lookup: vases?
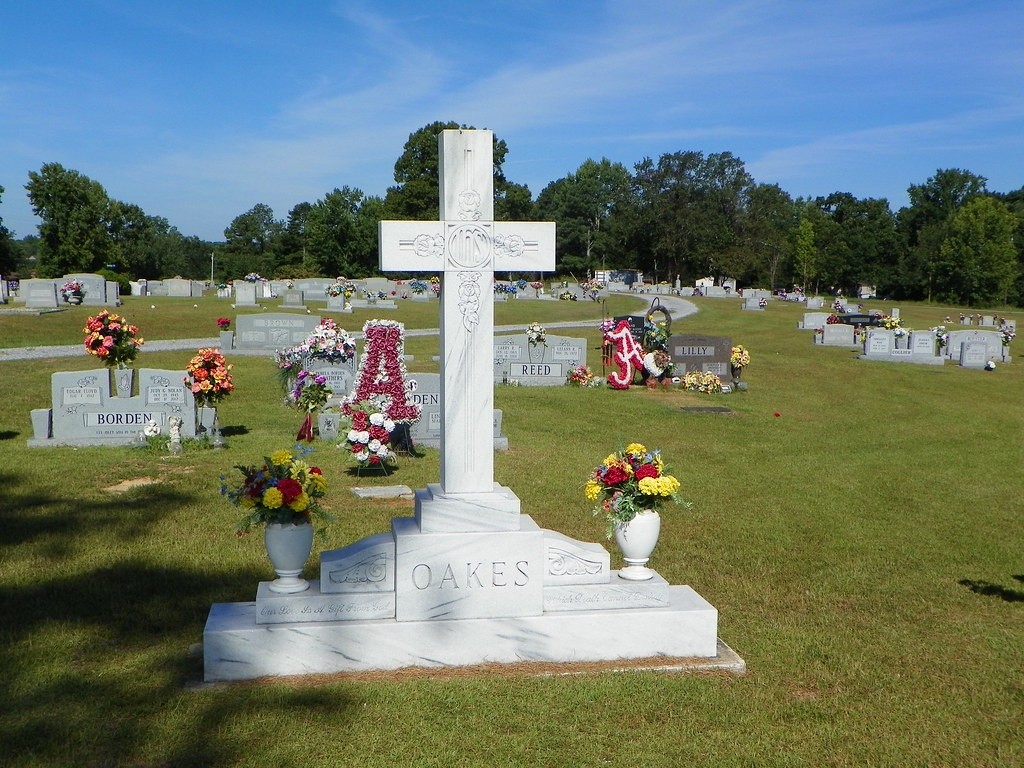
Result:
[731,365,741,378]
[197,407,217,436]
[68,296,80,306]
[939,346,947,356]
[30,408,53,439]
[615,507,660,580]
[220,330,234,350]
[896,337,909,349]
[318,413,340,440]
[114,369,134,397]
[854,335,862,343]
[286,379,297,391]
[1002,346,1010,357]
[264,522,315,594]
[814,334,823,344]
[530,342,545,364]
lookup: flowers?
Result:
[324,276,357,311]
[219,284,226,288]
[431,276,440,295]
[82,310,146,370]
[409,278,428,293]
[401,292,408,299]
[391,290,396,296]
[580,278,605,304]
[244,273,268,283]
[286,281,294,289]
[944,316,954,323]
[529,281,544,289]
[218,444,337,543]
[661,281,668,285]
[759,297,767,306]
[565,361,593,388]
[779,288,787,297]
[987,361,997,370]
[681,370,722,395]
[826,314,843,324]
[271,317,356,391]
[959,313,982,320]
[794,286,805,295]
[814,328,824,334]
[929,326,949,347]
[339,319,423,466]
[494,277,527,295]
[59,279,84,296]
[217,318,231,329]
[598,315,680,391]
[873,313,913,338]
[996,317,1017,346]
[579,438,695,542]
[362,289,387,300]
[833,300,845,312]
[729,345,751,369]
[560,291,578,301]
[854,322,871,342]
[182,347,234,407]
[523,321,548,347]
[281,371,332,413]
